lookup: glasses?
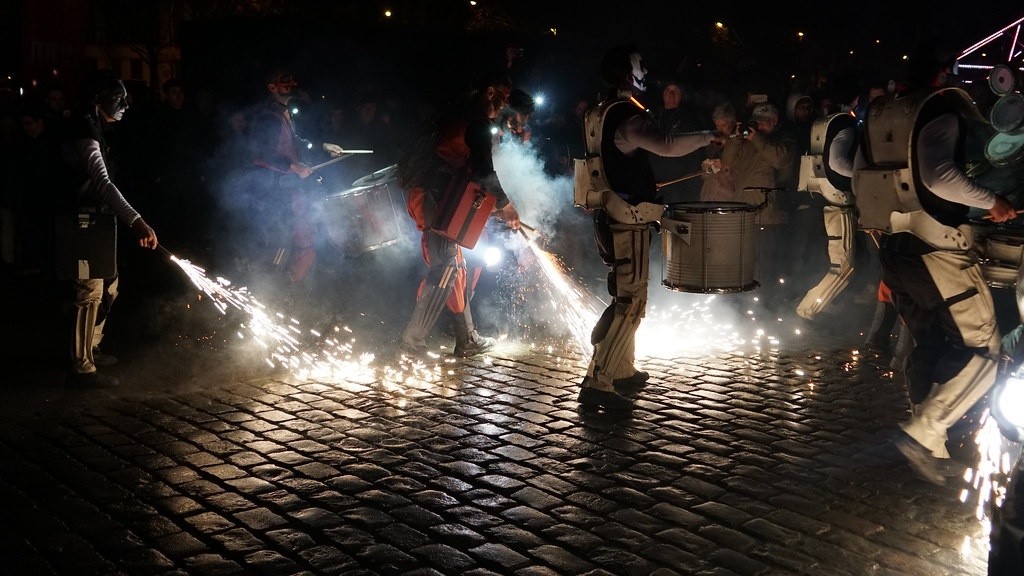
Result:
[796,105,811,111]
[22,120,37,128]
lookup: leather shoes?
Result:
[580,387,633,414]
[889,429,947,487]
[913,453,971,482]
[612,369,649,387]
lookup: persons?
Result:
[852,38,1003,489]
[983,262,1024,576]
[575,43,730,413]
[858,228,916,374]
[398,74,522,359]
[0,0,1024,311]
[64,76,160,391]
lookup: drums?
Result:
[315,165,418,257]
[965,215,1023,288]
[663,201,763,295]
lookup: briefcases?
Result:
[430,174,497,250]
[10,207,119,285]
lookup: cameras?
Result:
[736,121,759,140]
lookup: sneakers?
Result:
[395,343,447,367]
[455,332,498,357]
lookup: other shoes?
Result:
[69,373,120,390]
[90,354,119,368]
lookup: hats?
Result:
[256,70,297,89]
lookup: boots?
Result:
[866,301,896,359]
[891,325,914,371]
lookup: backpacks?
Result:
[396,119,446,230]
